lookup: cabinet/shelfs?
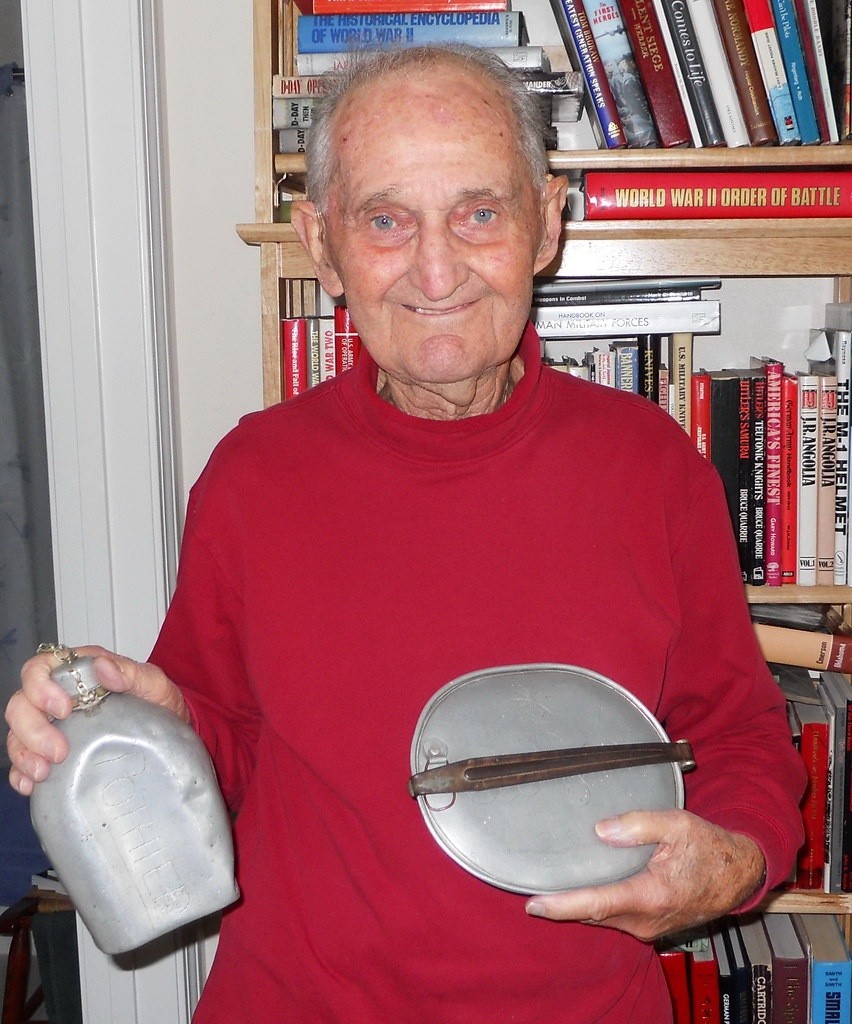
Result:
[236,0,852,1024]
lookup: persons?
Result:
[4,39,806,1024]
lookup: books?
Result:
[265,0,852,1024]
[26,868,67,894]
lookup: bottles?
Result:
[31,657,242,955]
